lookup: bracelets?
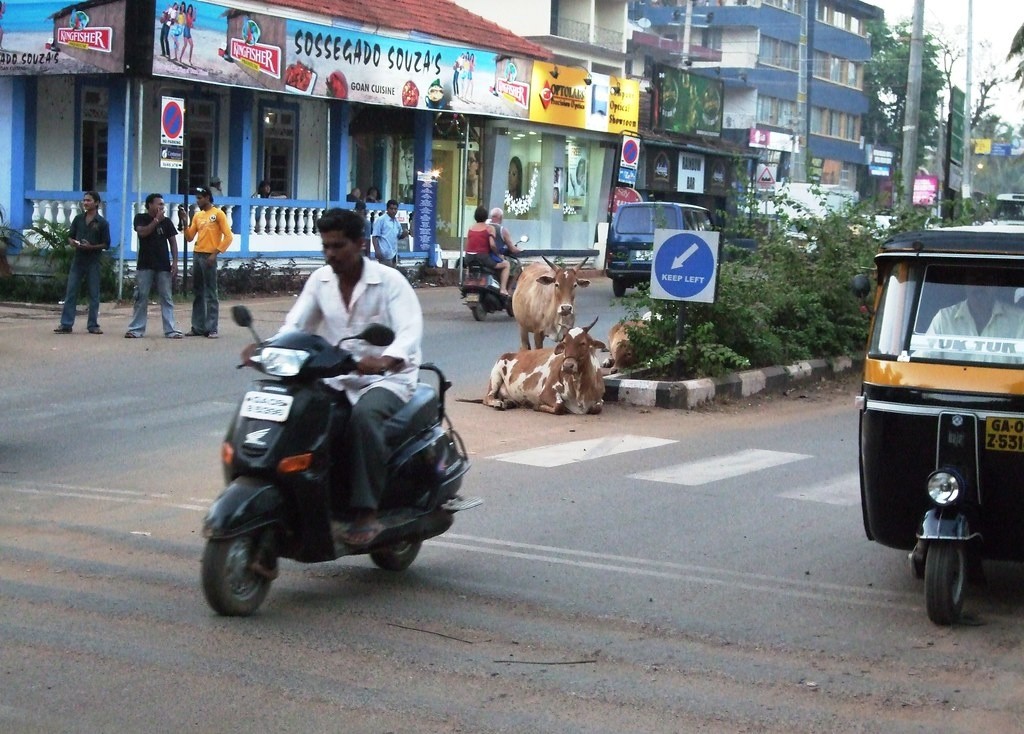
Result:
[154,218,159,224]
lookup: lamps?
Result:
[684,59,692,67]
[740,74,746,80]
[611,76,620,93]
[714,66,720,73]
[568,63,593,85]
[550,65,559,79]
[629,75,656,93]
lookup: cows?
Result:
[509,253,592,350]
[454,315,609,416]
[600,318,665,375]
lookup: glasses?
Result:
[196,188,207,193]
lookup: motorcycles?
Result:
[852,227,1024,625]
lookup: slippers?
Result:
[343,524,384,545]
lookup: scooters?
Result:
[201,305,485,614]
[458,234,531,322]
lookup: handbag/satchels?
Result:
[490,252,503,263]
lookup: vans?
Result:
[603,201,718,297]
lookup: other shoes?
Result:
[174,333,183,339]
[54,325,72,334]
[124,333,136,338]
[185,330,195,336]
[208,331,218,339]
[91,328,103,334]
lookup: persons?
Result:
[923,266,1024,339]
[508,156,522,200]
[554,188,559,205]
[179,187,234,338]
[125,194,182,339]
[355,202,371,258]
[347,189,361,202]
[54,190,111,334]
[372,199,409,267]
[488,208,523,263]
[179,5,196,66]
[453,52,475,102]
[366,188,380,203]
[467,206,511,297]
[160,2,178,58]
[208,176,223,197]
[172,2,185,61]
[240,208,423,547]
[253,181,286,199]
[466,151,480,198]
